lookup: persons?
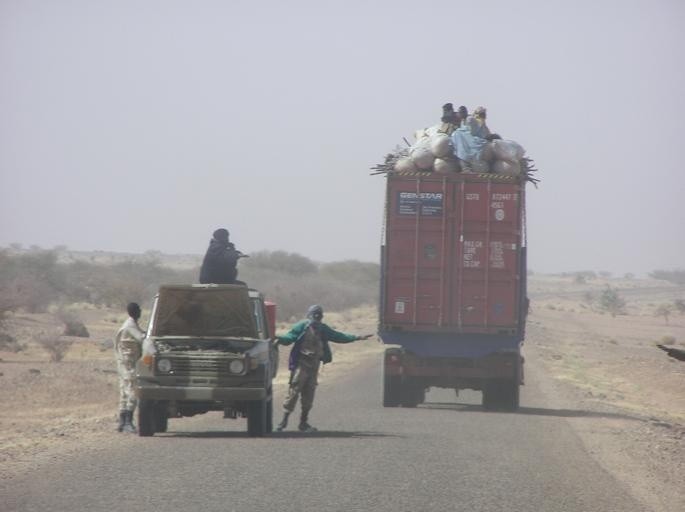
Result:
[272,304,375,433]
[467,106,490,136]
[198,228,250,286]
[441,104,461,125]
[457,106,469,119]
[112,300,145,434]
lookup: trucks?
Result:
[379,168,530,411]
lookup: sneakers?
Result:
[278,421,311,432]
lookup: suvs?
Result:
[134,280,280,437]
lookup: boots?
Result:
[117,410,136,433]
